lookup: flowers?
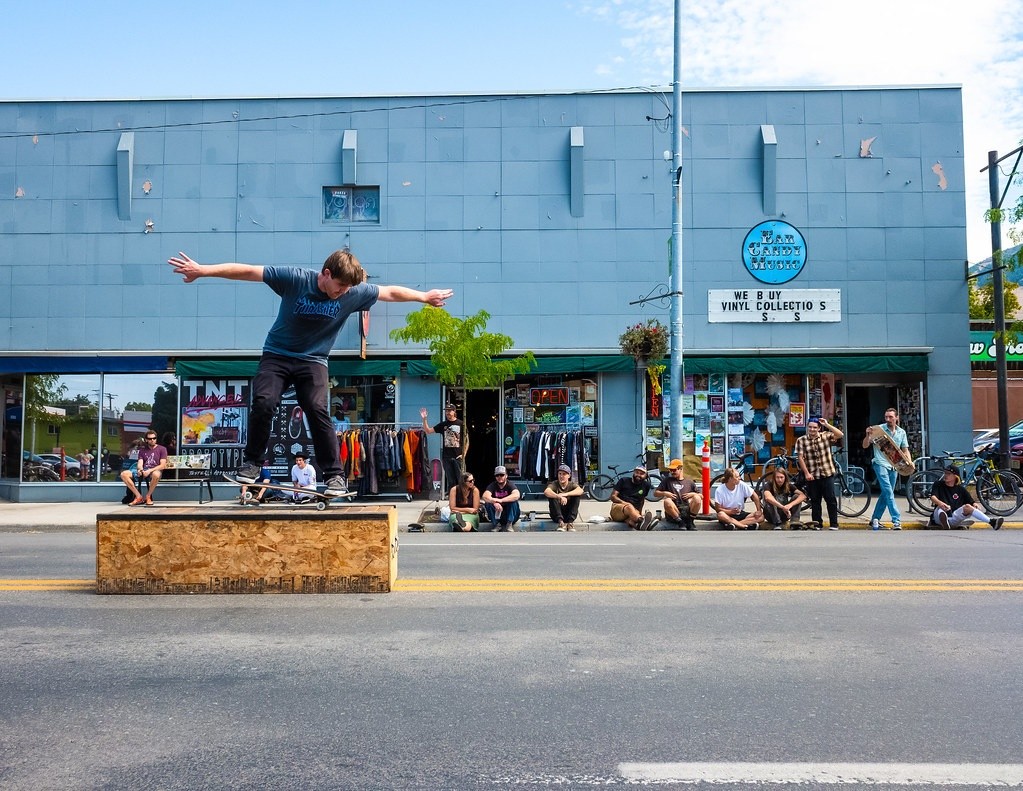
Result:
[619,320,666,360]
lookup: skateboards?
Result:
[915,518,975,530]
[801,521,820,531]
[407,523,426,532]
[868,425,915,476]
[790,523,803,530]
[519,510,550,522]
[222,472,358,512]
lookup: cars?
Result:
[22,449,139,482]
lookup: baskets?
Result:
[992,452,1010,470]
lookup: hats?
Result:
[667,459,683,469]
[557,465,572,474]
[634,465,646,474]
[293,452,309,460]
[494,466,508,474]
[808,418,818,422]
[442,404,457,410]
[946,466,961,475]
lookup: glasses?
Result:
[465,479,475,483]
[636,471,645,475]
[146,437,156,440]
[495,474,506,477]
[669,468,680,472]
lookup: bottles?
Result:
[645,509,649,514]
[736,503,741,515]
[140,470,143,481]
[873,519,879,531]
[435,500,439,515]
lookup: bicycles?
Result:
[708,441,872,517]
[904,417,1023,518]
[588,450,664,502]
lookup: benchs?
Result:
[132,454,215,503]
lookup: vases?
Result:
[636,338,654,355]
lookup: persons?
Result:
[167,250,453,495]
[863,408,911,530]
[483,466,521,532]
[449,472,480,532]
[101,443,110,476]
[74,449,94,480]
[762,467,806,530]
[793,418,845,530]
[239,468,273,503]
[88,444,97,478]
[162,432,177,456]
[931,465,1005,530]
[654,458,703,531]
[120,430,167,505]
[544,465,583,531]
[420,404,469,490]
[715,468,764,530]
[292,452,316,504]
[610,465,659,531]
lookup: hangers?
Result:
[331,423,424,436]
[527,420,583,432]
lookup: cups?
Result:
[656,510,661,521]
[521,520,531,532]
[539,521,545,531]
[530,511,535,522]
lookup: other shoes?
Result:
[635,511,902,530]
[456,512,467,527]
[938,512,951,530]
[557,524,566,531]
[507,523,514,532]
[129,497,154,505]
[452,522,462,532]
[491,523,502,532]
[989,517,1004,530]
[567,523,574,531]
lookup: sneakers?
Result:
[324,475,349,496]
[235,461,261,484]
